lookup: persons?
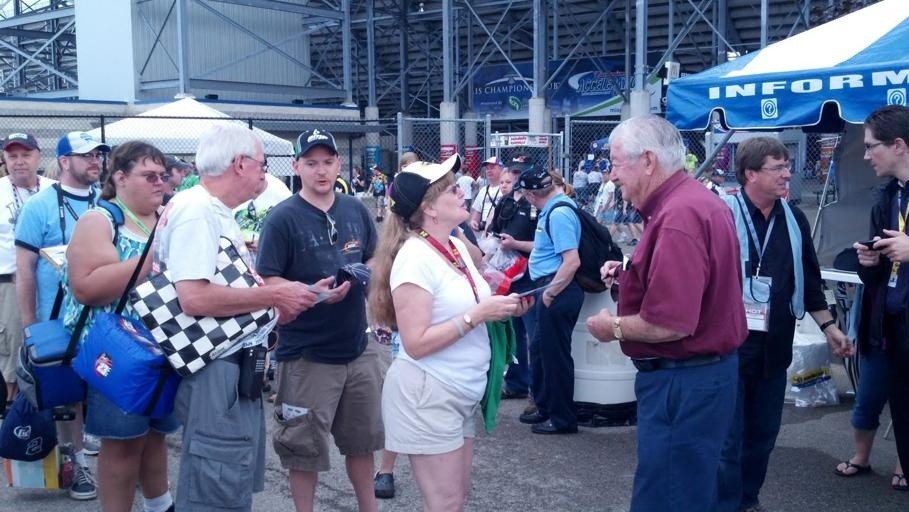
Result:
[1,124,586,512]
[717,136,855,511]
[831,105,909,492]
[569,145,731,246]
[585,114,749,511]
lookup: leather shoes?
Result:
[501,386,578,435]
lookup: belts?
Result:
[631,354,720,370]
[535,274,555,287]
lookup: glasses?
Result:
[64,152,101,161]
[233,155,268,172]
[863,143,882,152]
[144,176,169,183]
[756,164,790,173]
[445,184,460,194]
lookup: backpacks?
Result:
[546,201,624,292]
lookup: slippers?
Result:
[891,474,908,489]
[833,460,871,476]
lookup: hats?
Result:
[163,156,189,170]
[295,130,338,160]
[2,132,40,150]
[481,157,553,188]
[390,152,461,217]
[56,132,110,156]
[370,166,378,170]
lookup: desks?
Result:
[819,266,865,348]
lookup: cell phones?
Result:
[492,231,504,240]
[859,239,886,251]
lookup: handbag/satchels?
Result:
[0,312,180,462]
[237,346,265,402]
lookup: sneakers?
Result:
[374,470,395,497]
[68,433,100,500]
[618,232,638,246]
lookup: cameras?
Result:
[479,221,485,230]
